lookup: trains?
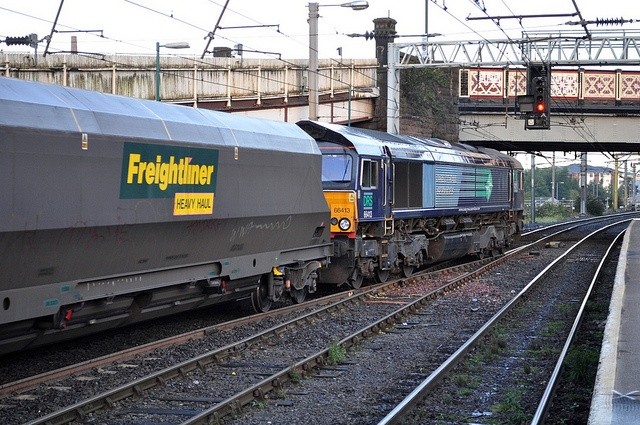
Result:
[0,70,527,359]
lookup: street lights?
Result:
[153,40,194,101]
[307,0,369,121]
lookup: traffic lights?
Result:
[523,62,550,130]
